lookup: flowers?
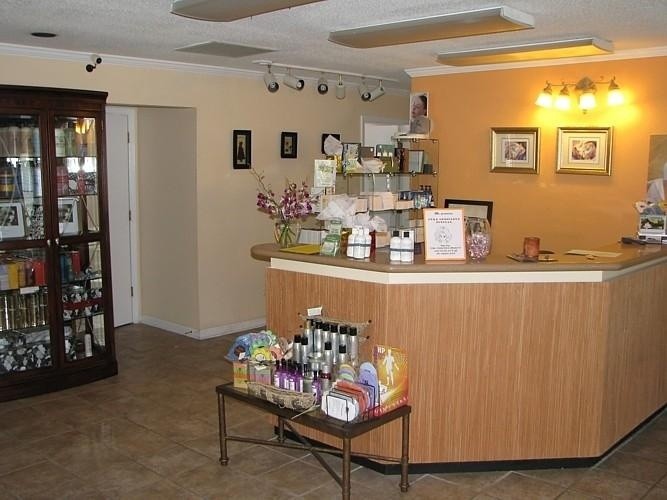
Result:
[251,167,321,250]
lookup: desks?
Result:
[215,380,411,500]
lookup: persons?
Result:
[2,208,15,226]
[64,206,72,222]
[473,222,482,235]
[410,94,427,120]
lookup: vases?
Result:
[274,219,302,249]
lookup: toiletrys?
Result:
[61,248,80,283]
[57,159,69,196]
[292,318,361,373]
[345,227,372,261]
[389,230,413,263]
[77,166,86,193]
[1,257,46,289]
[272,358,330,406]
[83,330,92,358]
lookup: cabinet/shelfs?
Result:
[0,83,118,405]
[335,138,438,231]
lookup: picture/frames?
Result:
[280,131,297,158]
[554,125,613,177]
[445,198,493,230]
[233,129,251,169]
[321,134,340,155]
[489,126,541,175]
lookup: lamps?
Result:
[327,6,536,49]
[436,39,614,67]
[536,75,624,109]
[261,62,386,103]
[170,0,324,22]
